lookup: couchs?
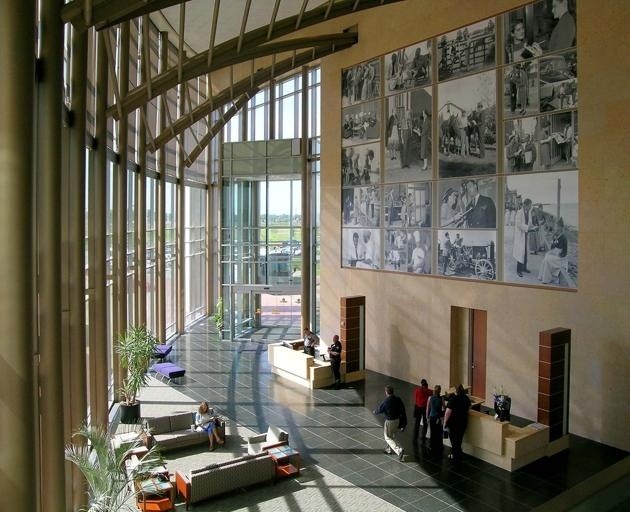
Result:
[141,408,225,452]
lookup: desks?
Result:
[263,441,301,482]
[135,475,173,512]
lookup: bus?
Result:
[258,246,293,286]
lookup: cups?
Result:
[191,424,195,432]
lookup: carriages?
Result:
[437,103,481,161]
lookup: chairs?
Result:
[247,424,289,456]
[125,454,170,492]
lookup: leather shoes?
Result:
[208,439,225,451]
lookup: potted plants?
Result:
[213,297,225,339]
[112,320,162,423]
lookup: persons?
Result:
[302,327,319,358]
[327,334,342,384]
[341,0,576,285]
[372,383,408,462]
[443,384,472,474]
[425,385,446,449]
[411,378,433,441]
[195,401,224,453]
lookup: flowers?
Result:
[491,385,506,410]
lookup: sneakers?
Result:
[398,448,406,462]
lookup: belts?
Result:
[387,417,400,420]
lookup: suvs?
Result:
[446,244,496,281]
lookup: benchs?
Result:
[152,362,186,385]
[149,345,172,362]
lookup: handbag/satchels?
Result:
[397,408,407,431]
[443,430,449,439]
